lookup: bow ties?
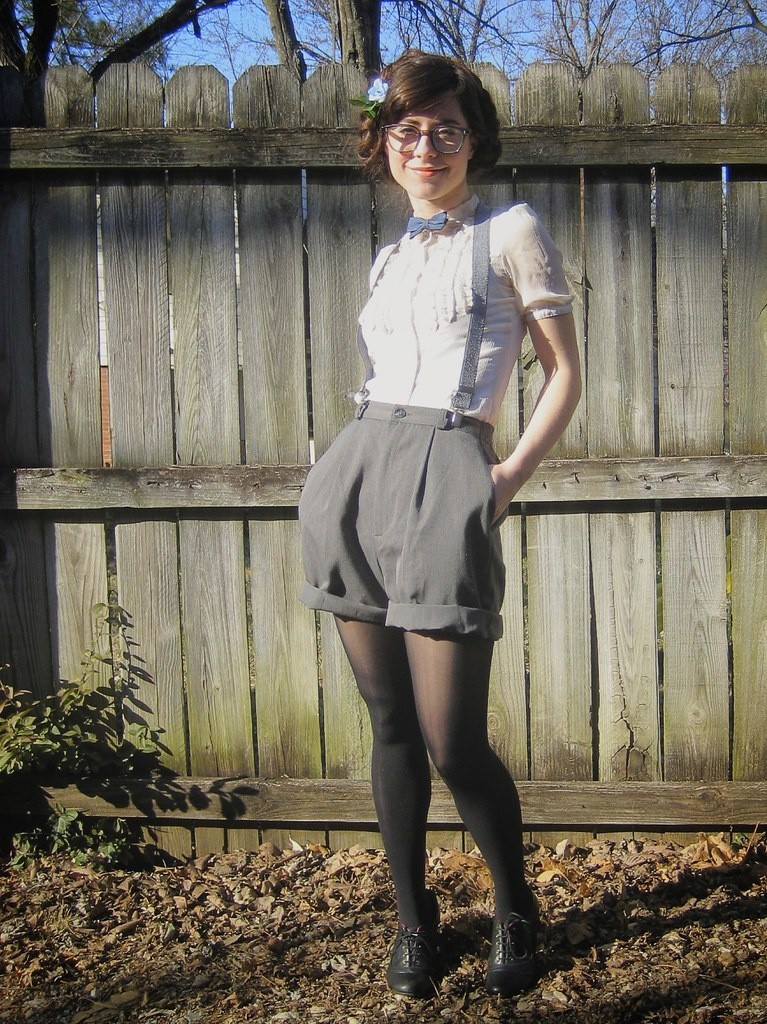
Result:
[407,211,448,240]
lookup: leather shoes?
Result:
[382,889,439,997]
[486,883,540,997]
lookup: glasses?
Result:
[382,124,472,154]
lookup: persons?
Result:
[296,52,581,998]
